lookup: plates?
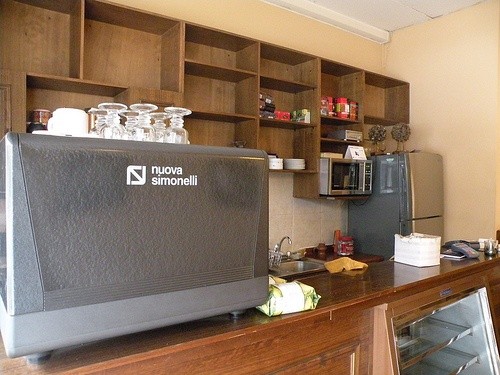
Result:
[284,159,306,170]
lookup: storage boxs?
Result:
[394,232,441,267]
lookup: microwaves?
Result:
[320,159,373,195]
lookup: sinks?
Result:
[280,257,328,276]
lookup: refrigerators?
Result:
[347,153,444,259]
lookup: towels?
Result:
[324,257,368,274]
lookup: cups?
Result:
[47,107,89,134]
[484,240,497,257]
[31,109,51,123]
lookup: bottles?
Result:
[27,119,44,133]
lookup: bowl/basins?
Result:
[268,158,284,170]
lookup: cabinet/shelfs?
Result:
[88,250,500,375]
[0,0,410,200]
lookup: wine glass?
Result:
[87,103,193,144]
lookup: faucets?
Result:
[278,235,293,254]
